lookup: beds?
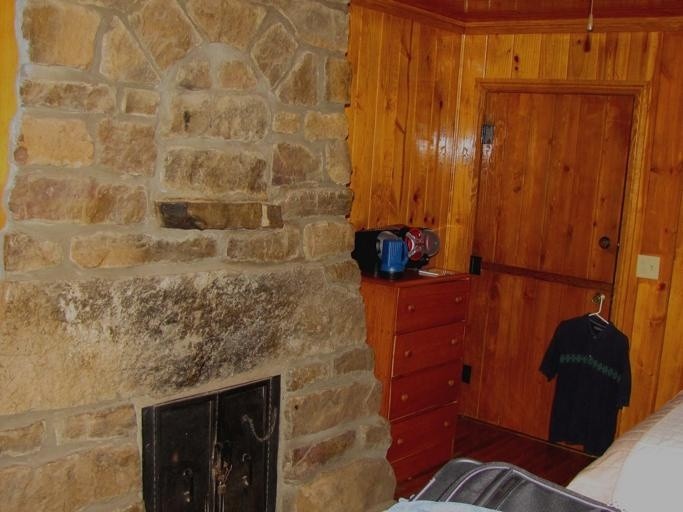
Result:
[567,388,683,512]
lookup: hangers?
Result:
[587,294,610,325]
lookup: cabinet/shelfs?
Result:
[358,278,469,500]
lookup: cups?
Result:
[382,239,407,276]
